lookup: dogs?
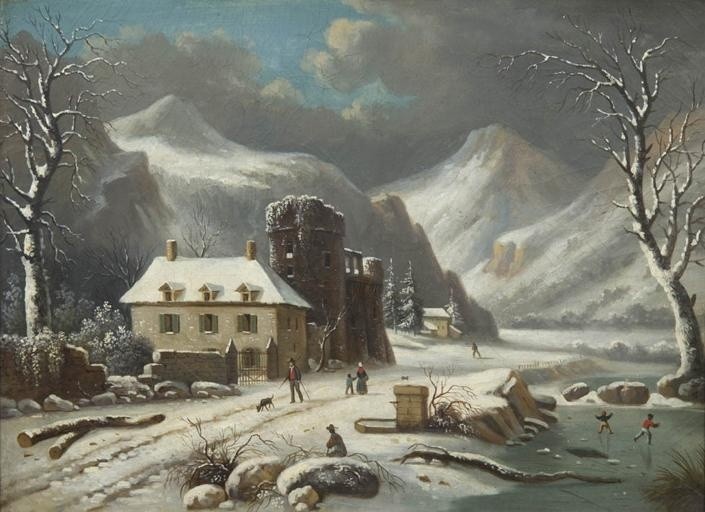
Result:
[255,394,275,413]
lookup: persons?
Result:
[472,341,481,359]
[284,355,305,405]
[325,423,347,459]
[355,360,369,395]
[593,408,615,436]
[345,373,357,394]
[632,413,662,444]
